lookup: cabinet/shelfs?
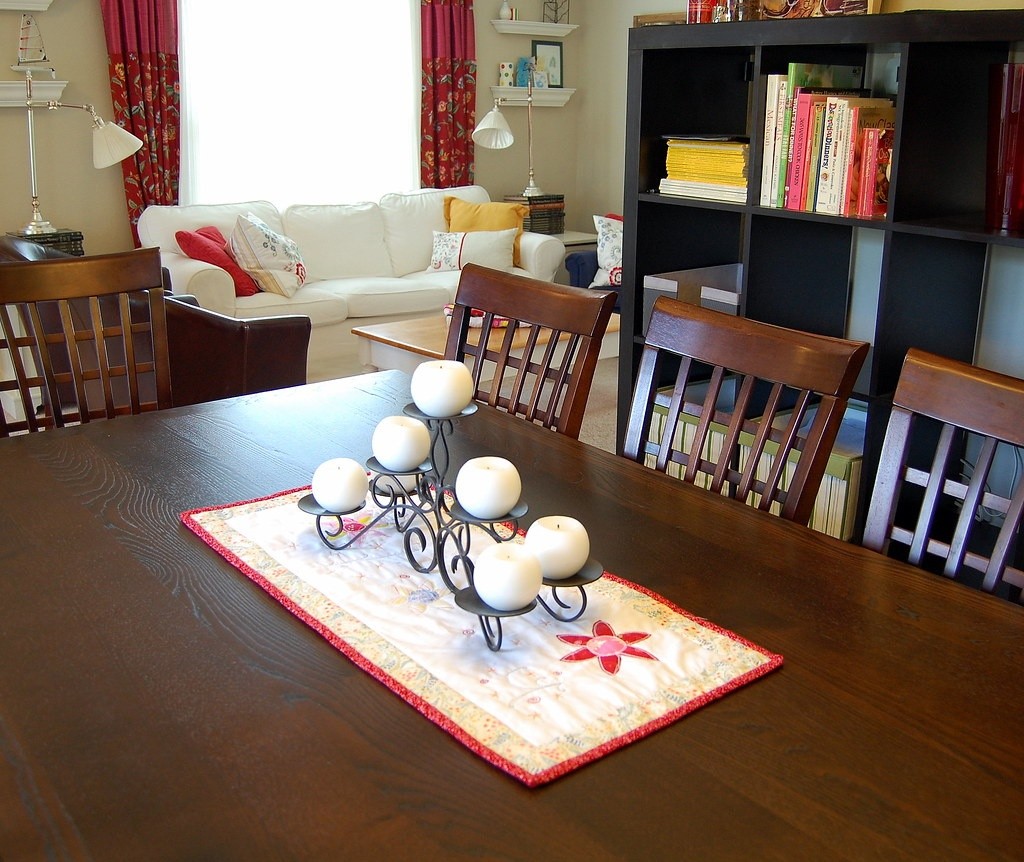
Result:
[490,18,580,107]
[613,5,1024,570]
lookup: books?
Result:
[760,63,894,218]
[659,137,748,205]
[505,196,565,234]
[5,228,84,256]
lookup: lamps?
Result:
[10,62,145,234]
[469,58,544,197]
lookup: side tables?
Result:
[551,230,598,287]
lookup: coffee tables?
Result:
[350,277,621,426]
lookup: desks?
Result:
[0,360,1024,862]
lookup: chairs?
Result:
[438,255,617,431]
[0,243,171,444]
[618,290,873,521]
[861,344,1024,593]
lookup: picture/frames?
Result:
[531,39,565,88]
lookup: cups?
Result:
[986,62,1024,231]
[712,6,727,23]
[499,62,514,86]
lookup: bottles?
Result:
[499,0,511,20]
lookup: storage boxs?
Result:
[640,262,743,337]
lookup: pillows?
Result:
[172,226,258,295]
[589,214,622,291]
[443,194,531,269]
[426,227,520,277]
[224,209,309,299]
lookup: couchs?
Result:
[565,211,622,314]
[136,180,566,382]
[0,232,325,402]
